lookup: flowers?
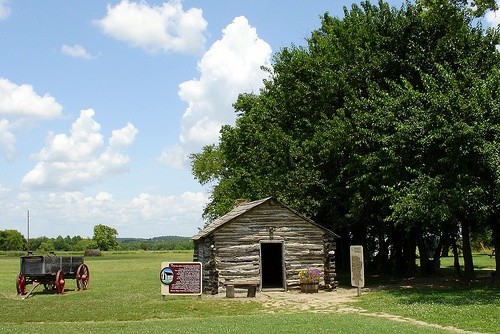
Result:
[298,267,321,284]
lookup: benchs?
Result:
[222,281,261,299]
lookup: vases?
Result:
[300,282,319,294]
